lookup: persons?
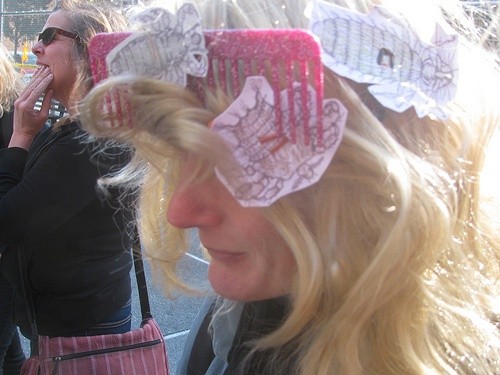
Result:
[0,42,50,375]
[78,1,500,375]
[0,1,140,356]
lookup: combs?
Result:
[87,32,326,153]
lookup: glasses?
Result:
[38,27,81,45]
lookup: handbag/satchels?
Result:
[20,318,170,375]
